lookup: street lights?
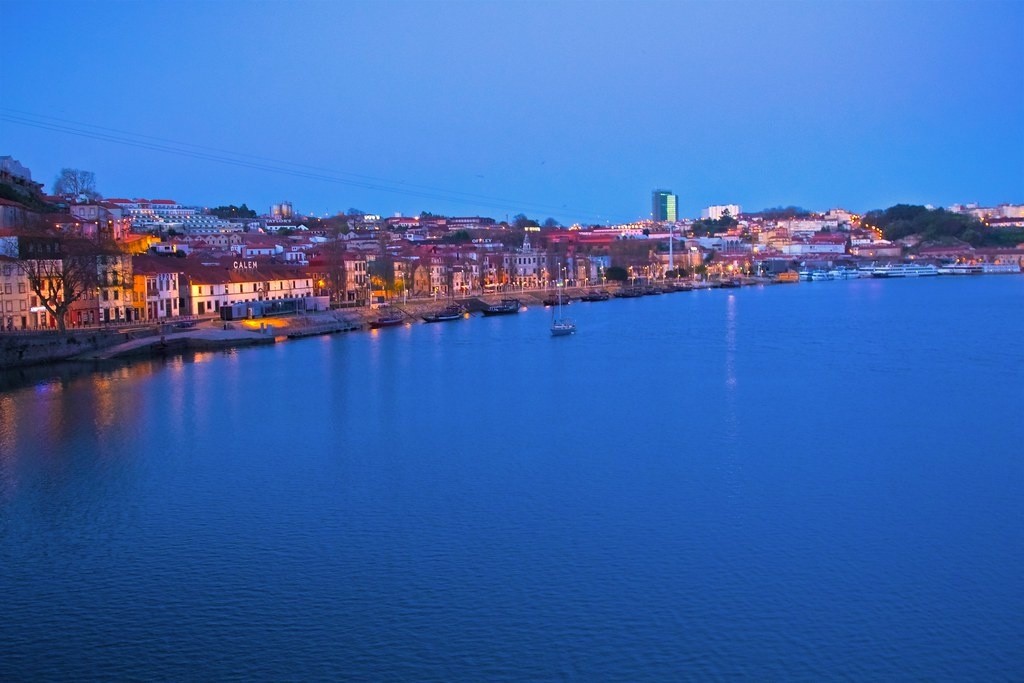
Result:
[560,266,568,289]
[540,268,547,292]
[630,266,635,287]
[399,273,407,306]
[599,265,605,287]
[363,274,372,309]
[676,264,680,283]
[558,263,561,291]
[460,268,467,299]
[520,267,525,295]
[646,265,650,283]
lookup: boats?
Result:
[578,290,611,301]
[542,292,572,306]
[796,262,1021,282]
[613,277,757,298]
[481,304,522,315]
[366,313,404,328]
[420,311,465,322]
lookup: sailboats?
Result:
[550,263,578,336]
